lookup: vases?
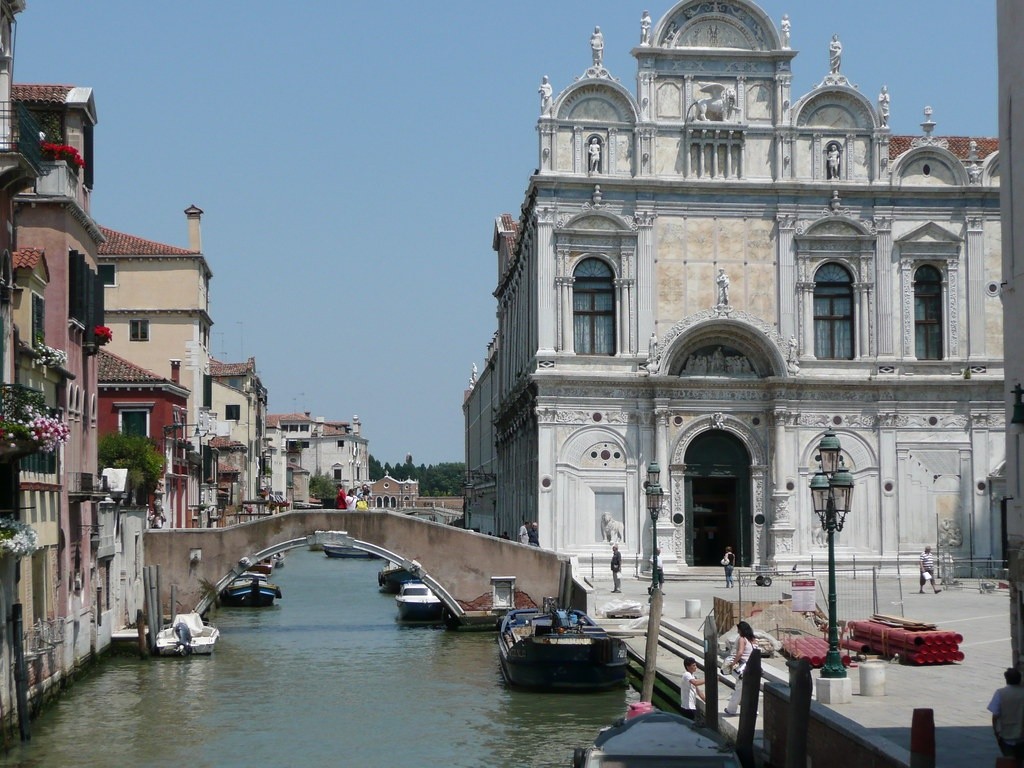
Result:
[46,150,79,174]
[0,444,37,460]
[95,334,107,345]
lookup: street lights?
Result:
[645,455,665,616]
[808,427,855,704]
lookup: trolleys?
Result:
[733,565,782,586]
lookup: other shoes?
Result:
[648,587,652,594]
[611,589,621,593]
[661,591,666,595]
[724,707,735,715]
[935,589,941,593]
[920,590,925,593]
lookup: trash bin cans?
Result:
[941,553,955,584]
[627,702,651,721]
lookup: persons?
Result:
[649,548,666,595]
[640,10,651,44]
[488,531,510,540]
[538,76,553,114]
[686,347,751,374]
[589,139,600,172]
[590,27,604,67]
[665,23,678,41]
[611,545,622,593]
[649,333,658,358]
[716,268,729,305]
[724,547,735,588]
[987,668,1024,757]
[725,622,759,716]
[919,546,942,594]
[789,335,797,359]
[830,35,842,75]
[781,15,791,50]
[827,145,840,179]
[681,657,705,720]
[519,521,539,547]
[714,412,723,428]
[879,86,890,127]
[336,484,370,509]
[754,24,763,41]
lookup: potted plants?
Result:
[97,429,165,506]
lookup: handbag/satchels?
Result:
[616,571,623,578]
[922,571,932,580]
[721,557,730,565]
[357,497,368,510]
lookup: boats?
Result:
[395,580,443,620]
[219,557,278,608]
[157,613,220,657]
[573,711,743,768]
[377,561,418,594]
[323,544,380,558]
[496,596,630,694]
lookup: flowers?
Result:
[39,140,86,169]
[0,515,39,562]
[0,404,71,454]
[95,325,112,344]
[35,341,67,368]
